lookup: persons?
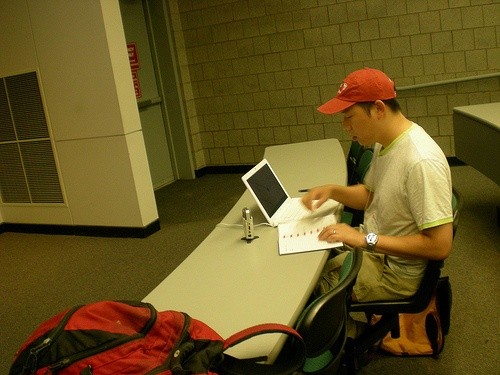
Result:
[300,69,452,302]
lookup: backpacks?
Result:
[7,298,306,375]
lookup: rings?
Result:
[334,230,336,234]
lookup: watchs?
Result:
[365,232,378,251]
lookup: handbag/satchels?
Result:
[373,276,453,361]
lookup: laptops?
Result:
[241,159,340,227]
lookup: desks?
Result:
[453,101,500,186]
[140,138,348,364]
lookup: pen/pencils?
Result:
[298,188,309,192]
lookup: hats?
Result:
[316,67,397,115]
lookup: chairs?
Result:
[292,142,464,375]
